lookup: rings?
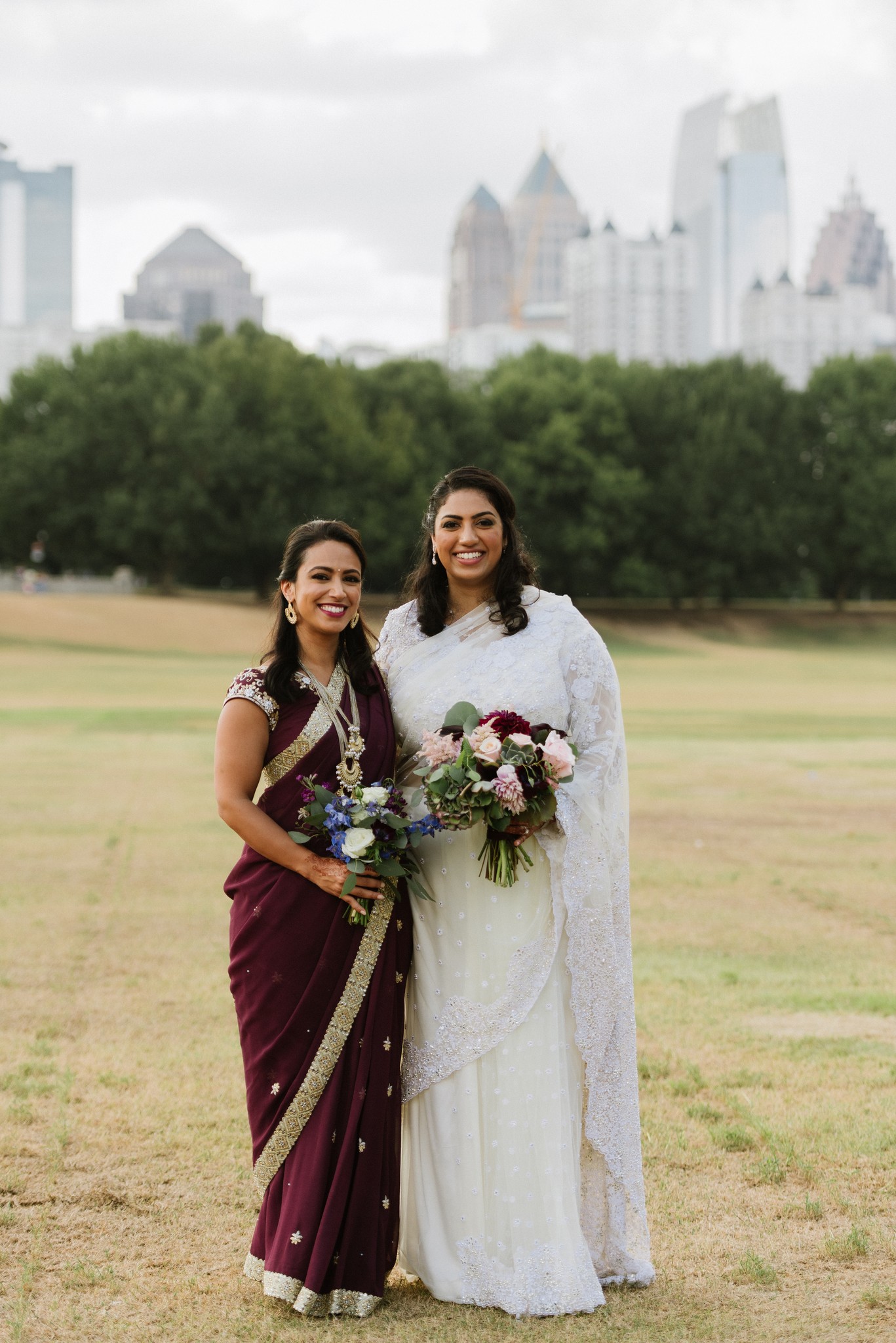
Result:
[522,825,529,835]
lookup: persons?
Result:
[212,520,409,1316]
[371,467,654,1318]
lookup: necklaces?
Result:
[448,603,483,636]
[297,654,367,800]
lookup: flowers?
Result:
[288,772,437,926]
[409,702,577,888]
